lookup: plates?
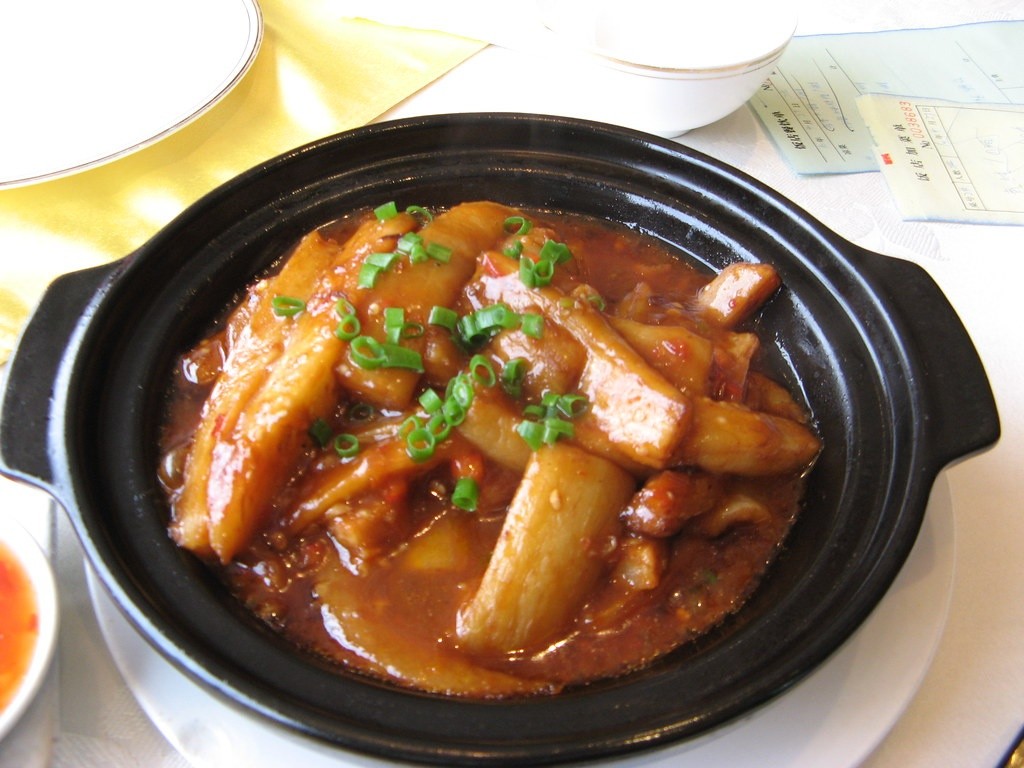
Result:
[86,443,961,767]
[0,510,62,756]
[0,1,265,190]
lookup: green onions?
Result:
[272,202,589,511]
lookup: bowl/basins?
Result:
[3,106,1002,768]
[550,1,797,148]
